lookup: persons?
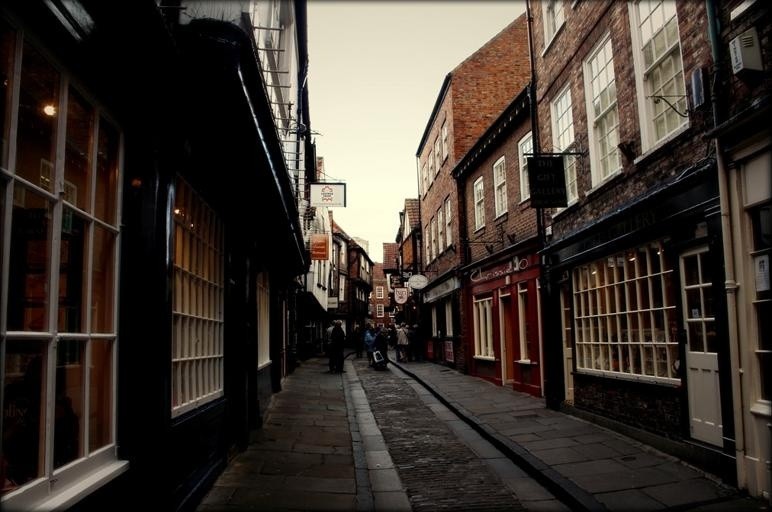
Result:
[329,320,347,374]
[353,318,420,368]
[324,320,336,370]
